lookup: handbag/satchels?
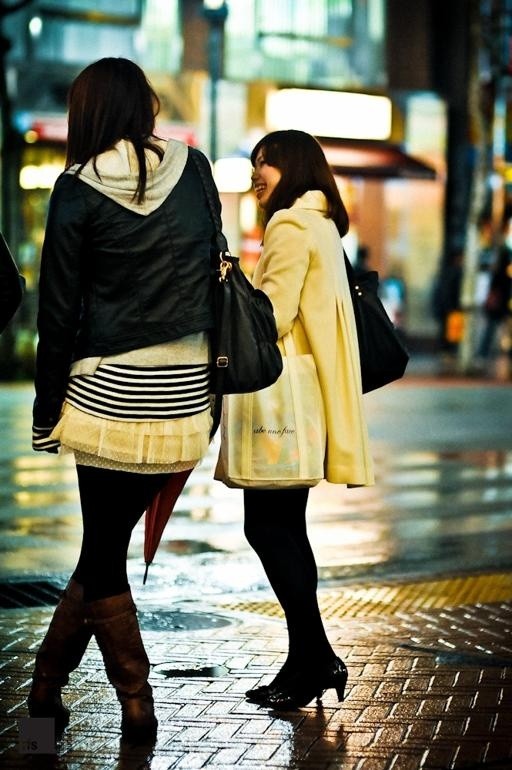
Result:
[343,248,409,394]
[210,249,282,394]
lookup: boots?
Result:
[81,591,156,744]
[27,578,93,726]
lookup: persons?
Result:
[27,59,229,731]
[219,129,379,711]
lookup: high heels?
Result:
[246,656,348,709]
